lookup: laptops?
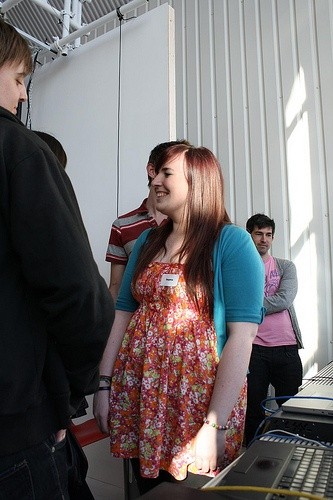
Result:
[211,440,333,500]
[282,384,333,417]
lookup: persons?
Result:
[0,20,116,500]
[93,144,263,495]
[242,214,303,446]
[105,140,196,305]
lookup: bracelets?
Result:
[94,386,110,390]
[98,375,111,383]
[202,416,230,430]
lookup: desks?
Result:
[267,407,333,444]
